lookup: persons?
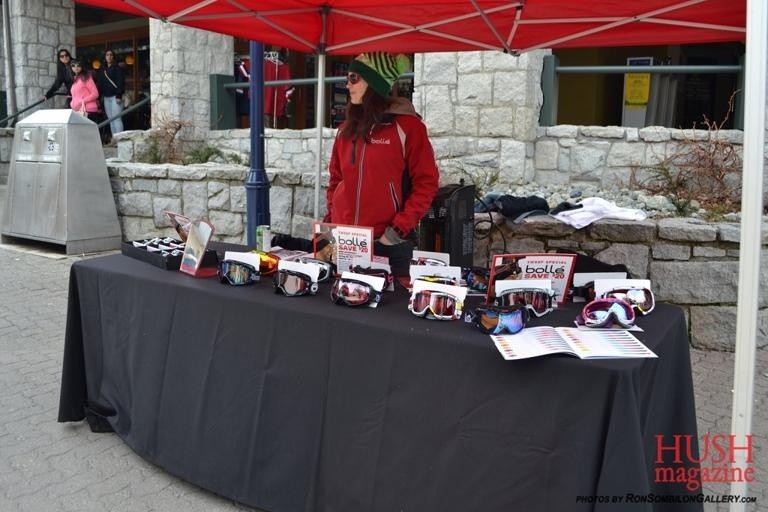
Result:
[323,52,439,272]
[42,49,125,147]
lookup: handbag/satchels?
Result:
[122,90,132,108]
[76,100,89,118]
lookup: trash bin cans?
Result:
[0,109,122,255]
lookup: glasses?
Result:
[250,249,281,275]
[582,297,636,325]
[499,288,549,317]
[409,257,458,320]
[604,286,655,316]
[349,72,360,85]
[271,269,311,298]
[217,259,255,287]
[71,64,80,67]
[474,305,527,336]
[350,267,389,292]
[462,264,490,291]
[61,54,68,58]
[329,278,372,307]
[292,256,330,282]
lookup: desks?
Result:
[66,242,687,512]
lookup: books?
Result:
[490,326,659,361]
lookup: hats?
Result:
[347,50,401,98]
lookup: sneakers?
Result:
[104,138,118,148]
